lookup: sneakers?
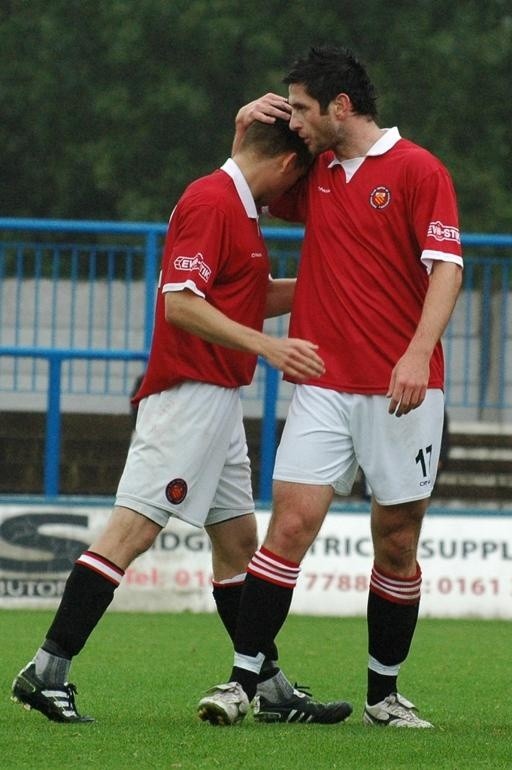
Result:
[195,681,249,726]
[13,661,94,724]
[253,688,352,725]
[362,695,436,729]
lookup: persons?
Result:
[196,45,463,728]
[9,100,353,724]
